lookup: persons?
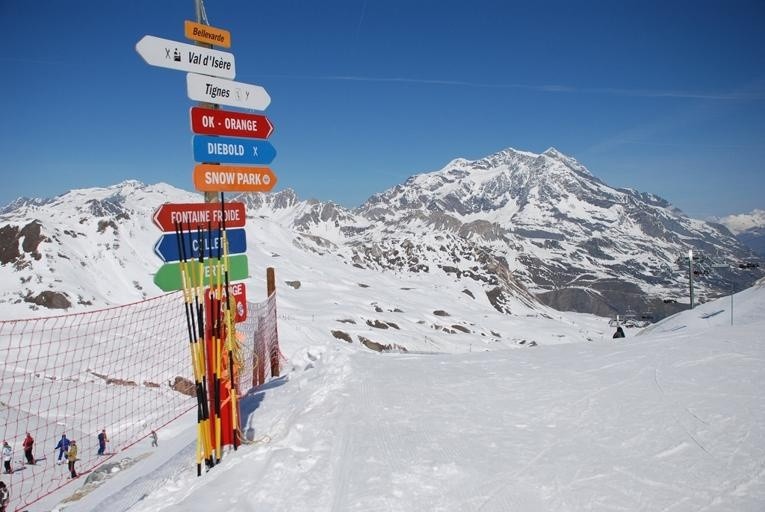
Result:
[64,439,78,479]
[0,440,14,475]
[22,432,35,465]
[0,481,10,511]
[149,429,158,448]
[96,428,110,455]
[54,434,71,465]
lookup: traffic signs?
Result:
[134,33,237,80]
[154,228,247,260]
[184,18,232,48]
[192,134,277,165]
[193,162,278,195]
[189,106,275,139]
[153,255,248,293]
[151,202,245,232]
[185,72,272,114]
